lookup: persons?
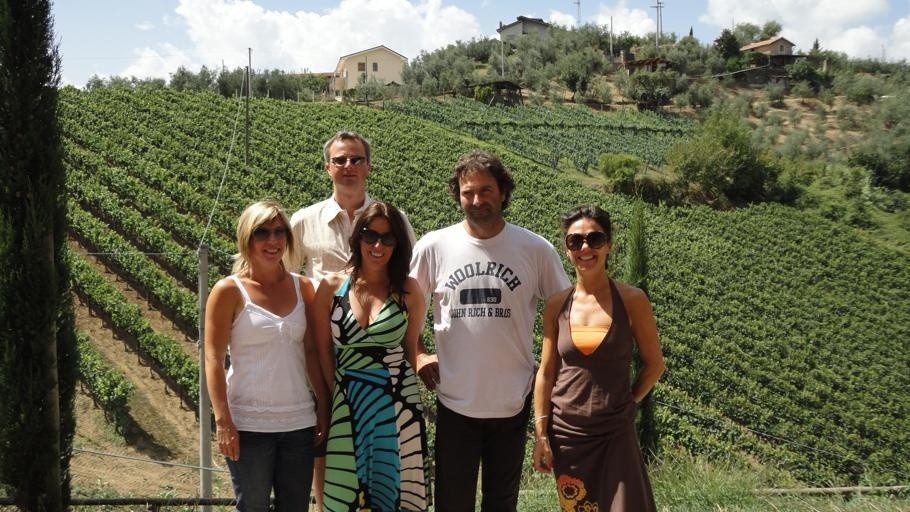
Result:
[201,201,333,511]
[282,131,419,294]
[405,149,574,511]
[532,204,666,511]
[310,200,433,511]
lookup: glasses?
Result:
[565,231,608,251]
[360,228,398,247]
[331,156,367,168]
[251,227,288,242]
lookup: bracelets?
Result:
[533,415,551,421]
[533,436,550,444]
